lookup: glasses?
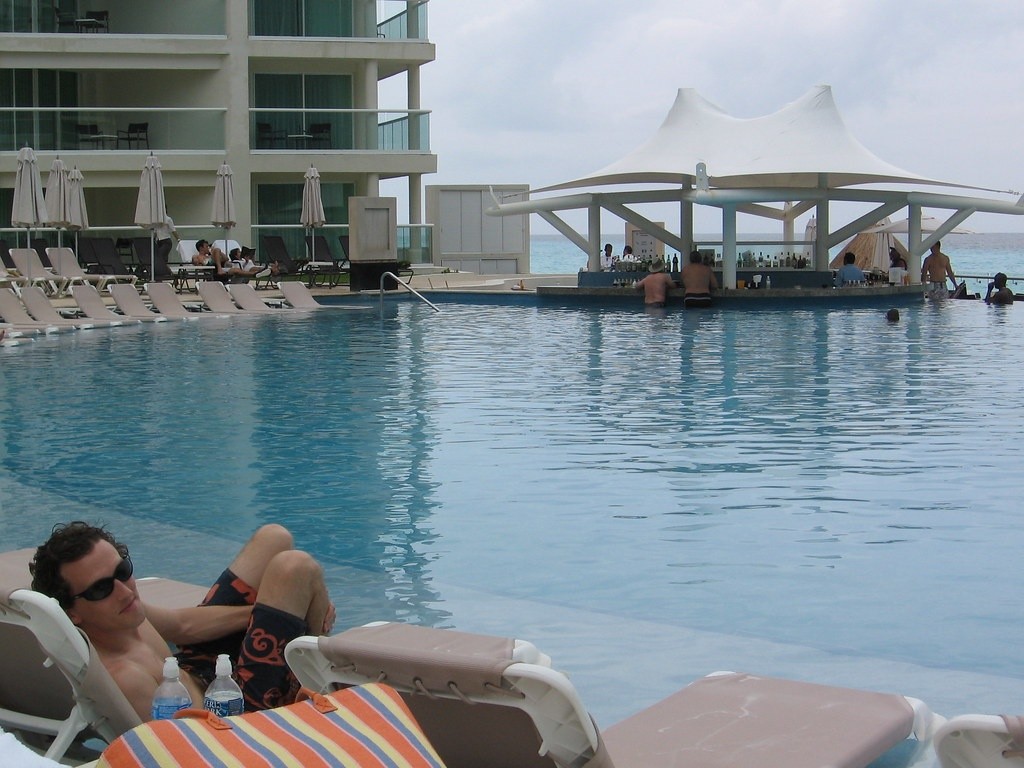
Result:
[72,556,133,601]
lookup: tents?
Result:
[829,214,909,268]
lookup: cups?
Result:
[904,274,910,285]
[737,280,745,289]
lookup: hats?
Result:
[890,246,903,259]
[649,257,665,272]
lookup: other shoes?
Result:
[255,269,272,279]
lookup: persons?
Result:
[152,199,182,279]
[680,250,719,311]
[889,246,905,287]
[191,238,272,278]
[25,514,336,729]
[921,240,959,304]
[622,246,639,263]
[633,258,677,316]
[984,272,1013,305]
[835,251,866,285]
[228,248,280,273]
[600,244,615,270]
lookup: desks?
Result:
[288,134,313,150]
[90,133,118,150]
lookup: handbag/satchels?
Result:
[96,681,450,768]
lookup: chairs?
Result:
[0,227,414,346]
[305,122,332,149]
[255,122,288,149]
[76,123,105,150]
[116,123,149,150]
[75,9,109,34]
[0,532,1023,768]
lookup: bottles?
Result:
[698,252,723,268]
[773,256,778,268]
[611,253,678,288]
[805,252,811,269]
[766,275,771,289]
[786,252,791,267]
[152,657,193,723]
[798,254,805,269]
[791,252,798,269]
[779,252,785,267]
[203,654,245,720]
[738,252,771,267]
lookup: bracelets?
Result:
[177,238,181,241]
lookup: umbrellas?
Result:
[299,163,326,263]
[12,140,48,248]
[45,155,69,249]
[64,166,90,262]
[135,151,165,280]
[211,160,237,257]
[857,213,975,251]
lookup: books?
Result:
[240,246,256,260]
